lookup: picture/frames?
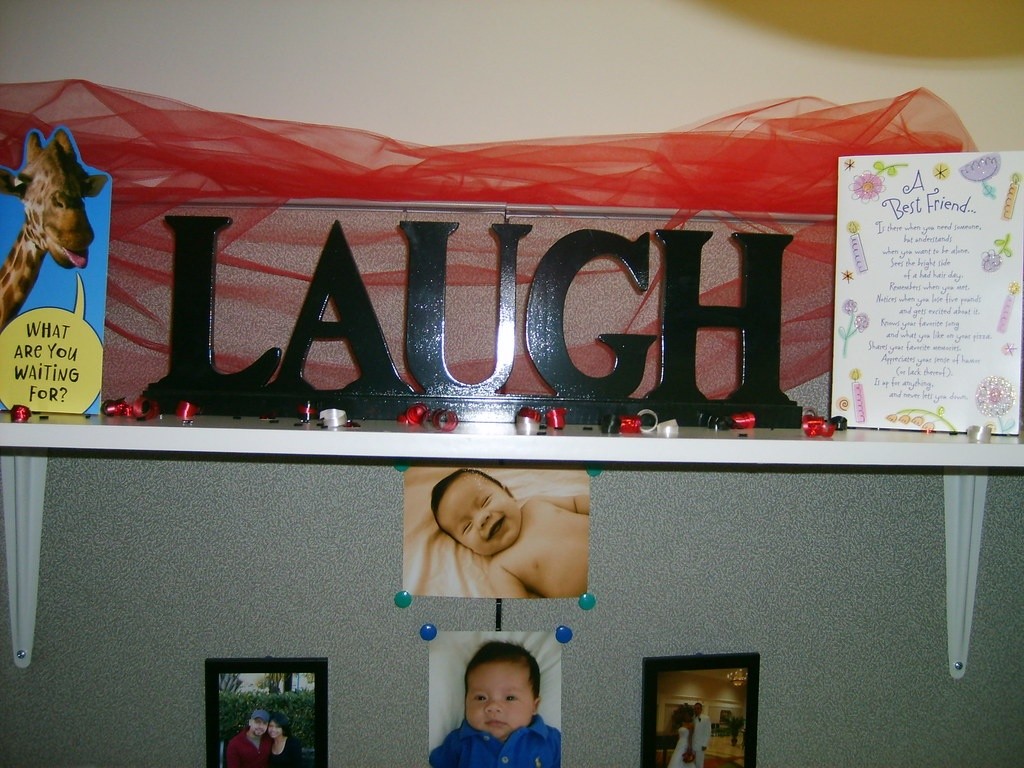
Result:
[204,657,329,768]
[641,653,760,768]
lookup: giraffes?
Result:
[0,126,110,337]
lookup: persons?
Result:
[667,703,711,768]
[429,641,562,768]
[226,709,303,768]
[430,469,590,599]
